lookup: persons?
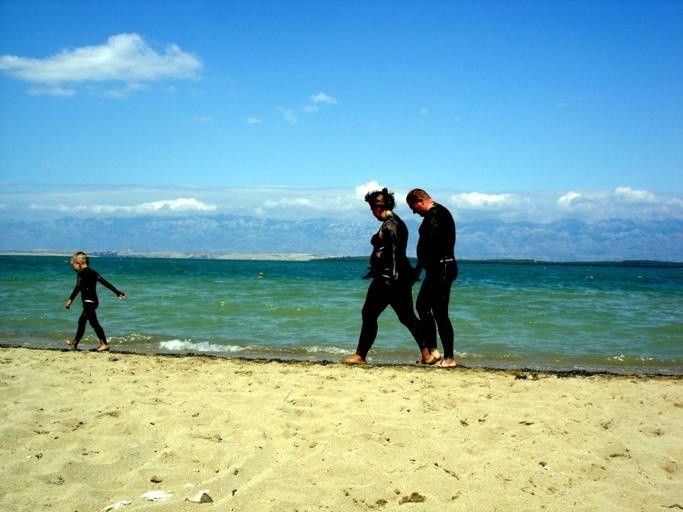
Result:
[62,251,128,353]
[405,188,459,368]
[339,186,435,366]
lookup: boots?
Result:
[65,339,77,348]
[97,340,110,351]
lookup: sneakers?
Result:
[343,356,367,364]
[416,350,456,367]
